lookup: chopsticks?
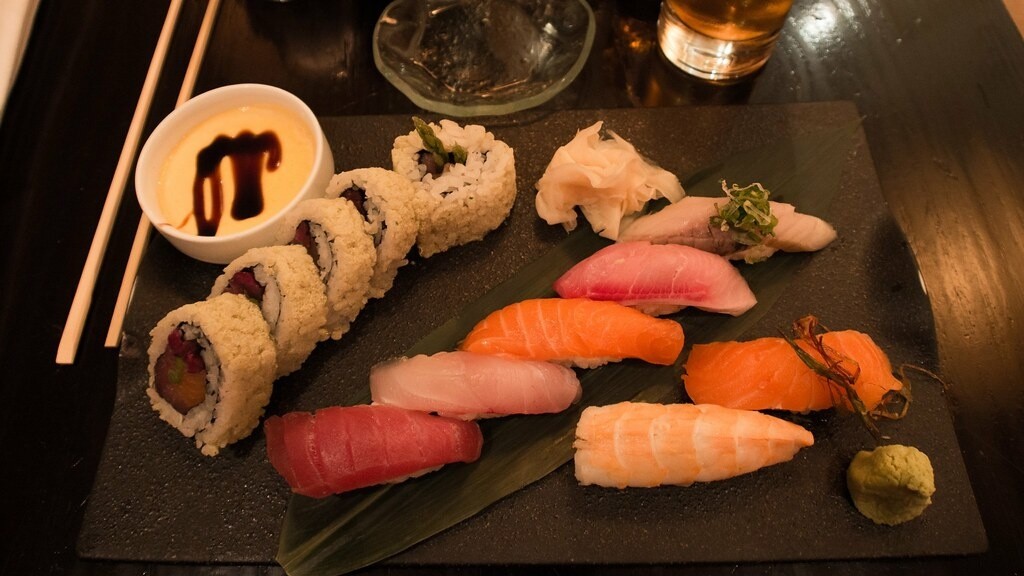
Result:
[55,0,220,365]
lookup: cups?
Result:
[657,0,792,87]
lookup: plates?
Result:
[373,0,597,117]
[79,103,989,564]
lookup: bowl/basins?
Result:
[134,85,335,265]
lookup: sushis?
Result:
[147,117,902,498]
